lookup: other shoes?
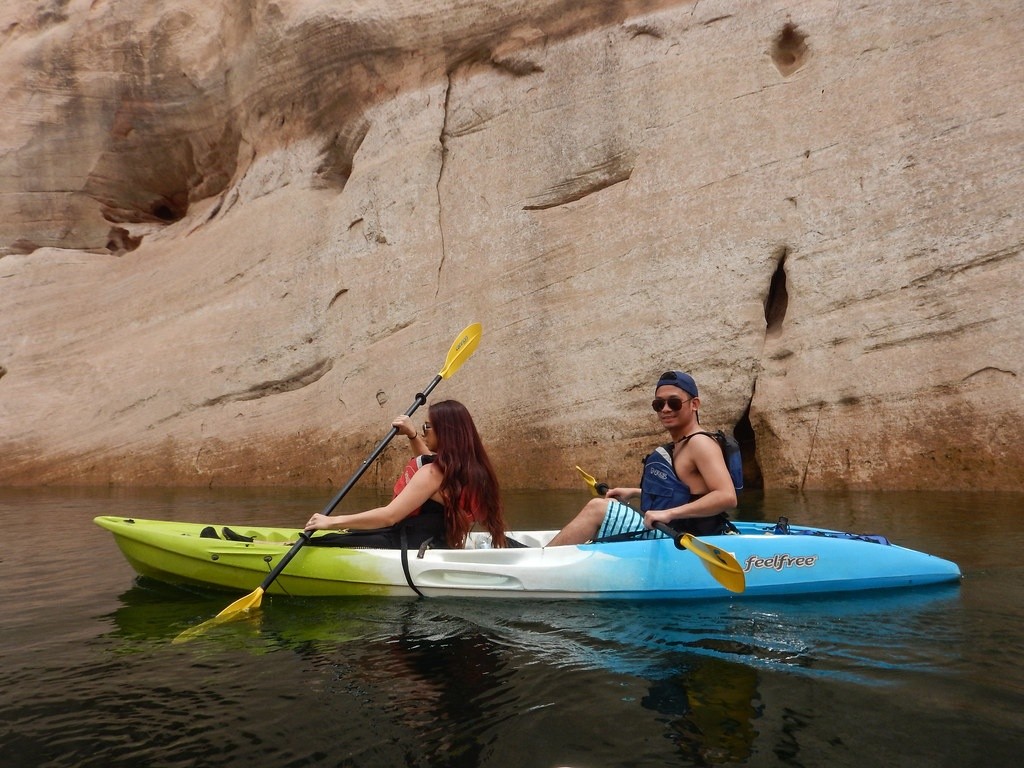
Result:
[503,536,532,548]
[201,526,223,541]
[223,527,256,543]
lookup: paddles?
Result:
[170,318,487,649]
[573,464,747,594]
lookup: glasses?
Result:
[421,423,435,434]
[651,398,694,412]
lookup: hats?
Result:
[655,371,699,398]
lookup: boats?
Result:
[99,517,964,599]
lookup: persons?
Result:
[544,370,743,548]
[200,400,507,549]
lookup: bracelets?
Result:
[408,432,417,440]
